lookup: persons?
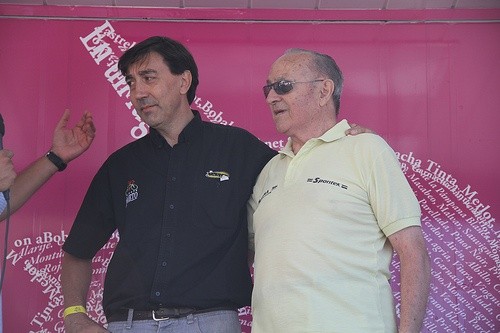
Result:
[0,108,97,333]
[60,35,379,333]
[246,47,432,333]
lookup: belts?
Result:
[107,304,238,322]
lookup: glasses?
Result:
[262,80,325,99]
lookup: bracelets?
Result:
[45,148,67,172]
[63,305,88,318]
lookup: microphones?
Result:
[0,113,9,200]
